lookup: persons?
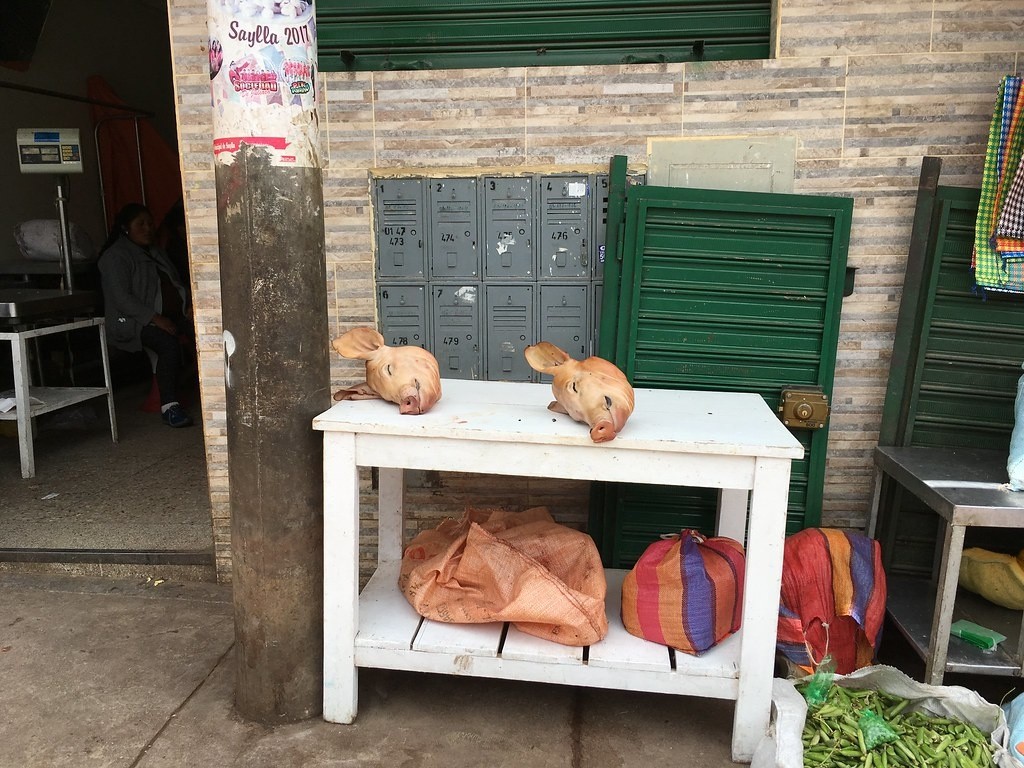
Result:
[97,205,200,427]
[167,205,190,287]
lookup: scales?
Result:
[0,126,85,334]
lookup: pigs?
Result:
[331,326,441,414]
[523,340,637,444]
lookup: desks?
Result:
[864,445,1024,688]
[310,377,805,765]
[0,316,119,479]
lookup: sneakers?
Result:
[163,404,190,426]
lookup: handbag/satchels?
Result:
[776,527,887,676]
[620,529,746,658]
